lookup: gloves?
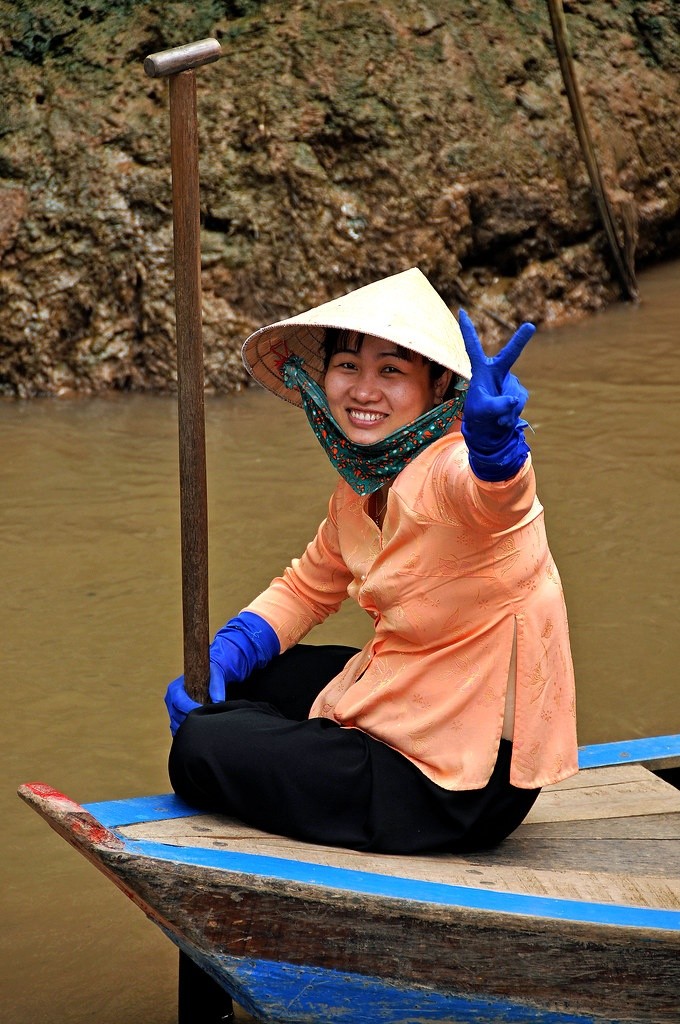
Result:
[165,612,278,735]
[455,308,538,483]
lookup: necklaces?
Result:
[374,496,387,526]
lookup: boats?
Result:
[16,718,679,1024]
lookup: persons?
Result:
[165,267,581,856]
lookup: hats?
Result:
[240,267,473,413]
